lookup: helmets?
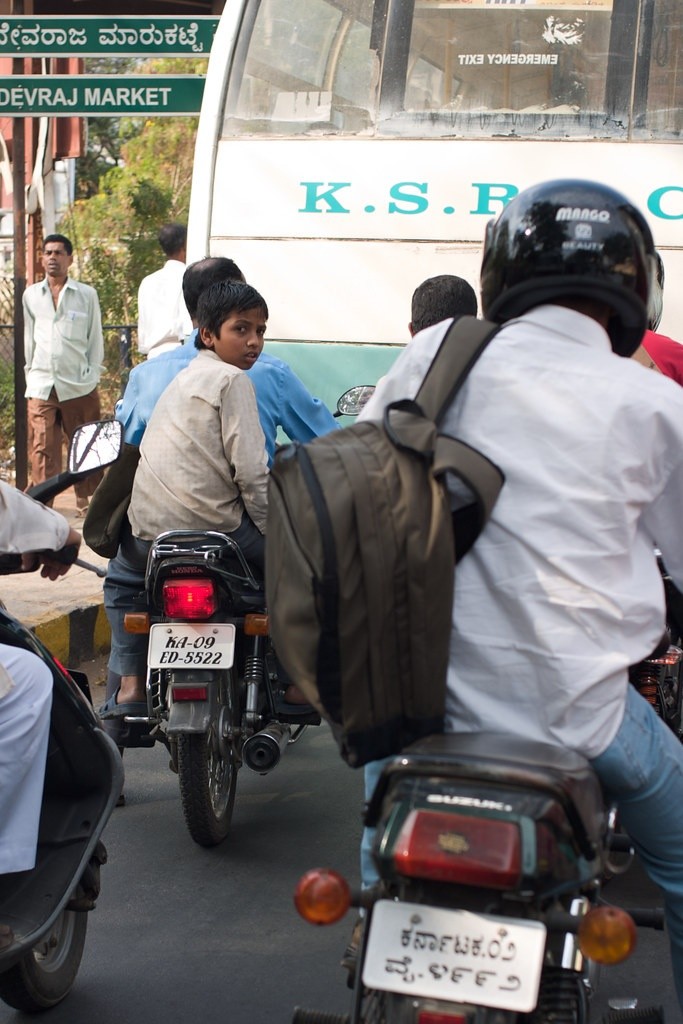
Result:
[481,179,665,358]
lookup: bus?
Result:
[185,0,683,556]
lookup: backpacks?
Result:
[268,314,506,769]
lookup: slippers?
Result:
[95,683,149,720]
[76,506,89,518]
[277,701,322,717]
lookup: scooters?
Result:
[0,418,121,1013]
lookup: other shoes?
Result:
[341,916,367,967]
[2,926,15,950]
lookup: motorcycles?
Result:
[114,384,376,848]
[291,549,683,1024]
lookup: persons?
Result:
[101,257,343,807]
[98,279,272,720]
[0,480,82,950]
[341,178,683,1024]
[21,234,105,518]
[408,275,477,336]
[136,225,193,361]
